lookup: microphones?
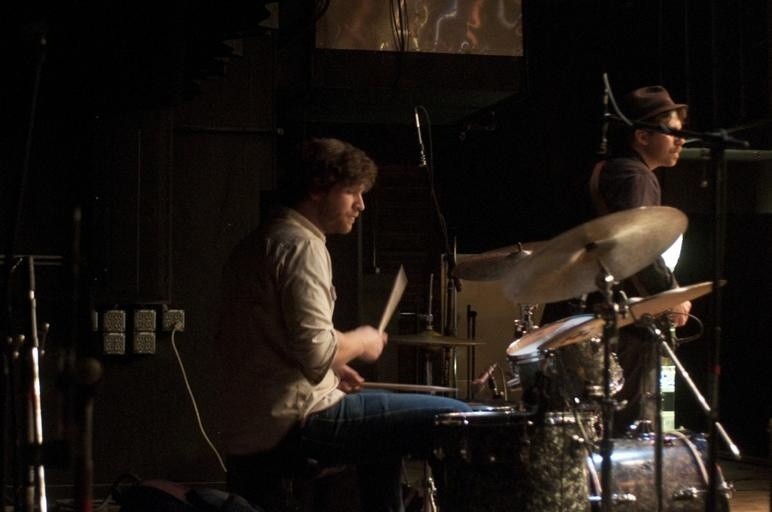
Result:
[412,106,432,182]
[592,92,612,157]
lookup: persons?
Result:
[219,136,474,512]
[588,85,692,440]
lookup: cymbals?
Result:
[454,240,549,281]
[388,331,487,350]
[538,279,727,350]
[500,206,689,303]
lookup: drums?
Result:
[430,410,600,512]
[506,313,626,409]
[589,419,736,511]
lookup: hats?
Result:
[607,86,690,129]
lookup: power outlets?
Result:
[136,309,157,331]
[135,332,155,356]
[103,309,126,333]
[164,309,185,332]
[104,331,126,355]
